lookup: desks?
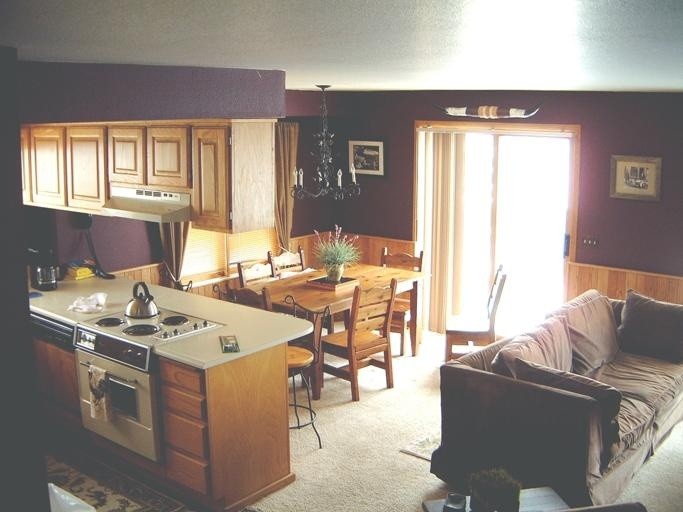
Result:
[244,262,423,403]
[422,485,571,512]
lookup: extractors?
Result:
[99,186,191,224]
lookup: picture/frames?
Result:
[349,140,384,176]
[609,153,662,202]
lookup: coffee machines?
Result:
[27,246,58,290]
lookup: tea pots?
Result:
[123,281,160,319]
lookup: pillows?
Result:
[488,290,683,467]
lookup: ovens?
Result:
[73,349,159,463]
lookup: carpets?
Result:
[399,430,441,461]
[43,451,259,512]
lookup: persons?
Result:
[469,465,521,512]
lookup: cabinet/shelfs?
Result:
[19,118,277,234]
[158,361,210,499]
[31,336,82,441]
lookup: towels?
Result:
[66,291,107,314]
[87,364,113,421]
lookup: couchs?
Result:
[429,296,683,507]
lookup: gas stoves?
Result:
[70,306,224,371]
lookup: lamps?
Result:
[289,85,361,200]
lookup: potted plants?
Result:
[310,225,358,281]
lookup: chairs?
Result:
[237,257,277,288]
[445,265,506,361]
[268,244,335,333]
[285,294,331,448]
[376,247,424,355]
[321,278,397,402]
[225,284,272,311]
